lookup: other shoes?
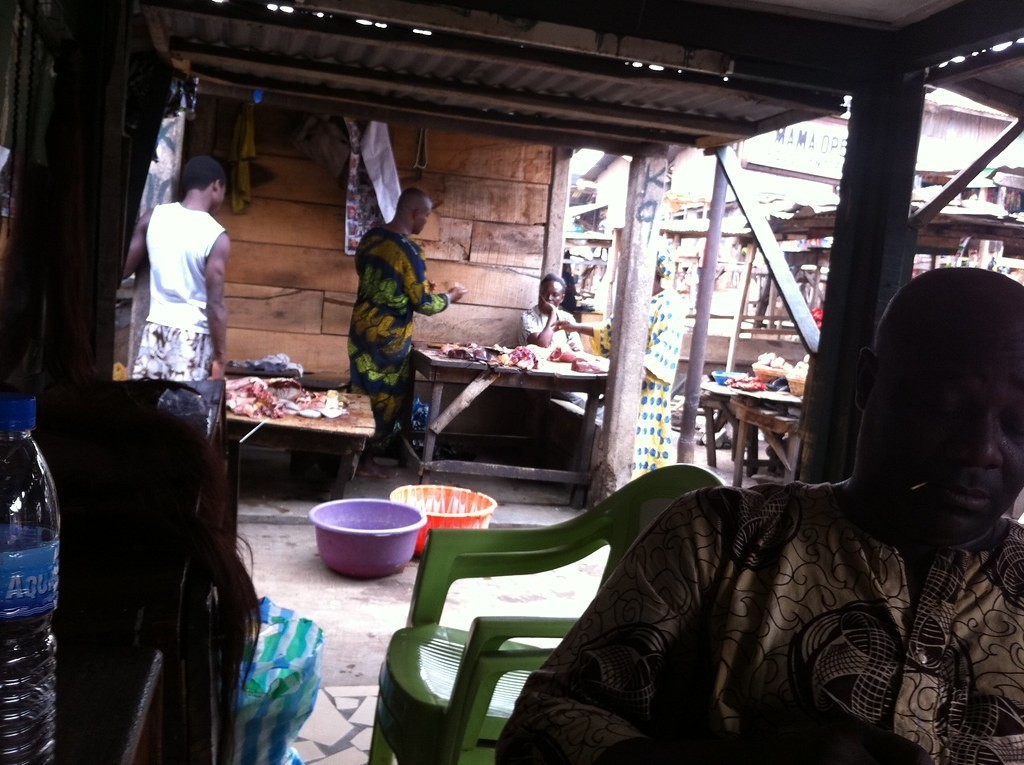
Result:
[358,467,400,480]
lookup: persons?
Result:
[121,155,232,382]
[348,188,468,478]
[496,268,1024,765]
[551,236,685,482]
[517,273,604,421]
[559,254,581,312]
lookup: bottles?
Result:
[0,390,59,765]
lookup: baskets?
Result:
[752,362,786,382]
[787,374,805,395]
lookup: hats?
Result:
[657,246,674,280]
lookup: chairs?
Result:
[366,462,722,765]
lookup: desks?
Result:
[729,390,804,487]
[226,393,377,503]
[412,347,610,510]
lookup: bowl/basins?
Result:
[389,484,498,557]
[711,371,739,385]
[308,497,428,577]
